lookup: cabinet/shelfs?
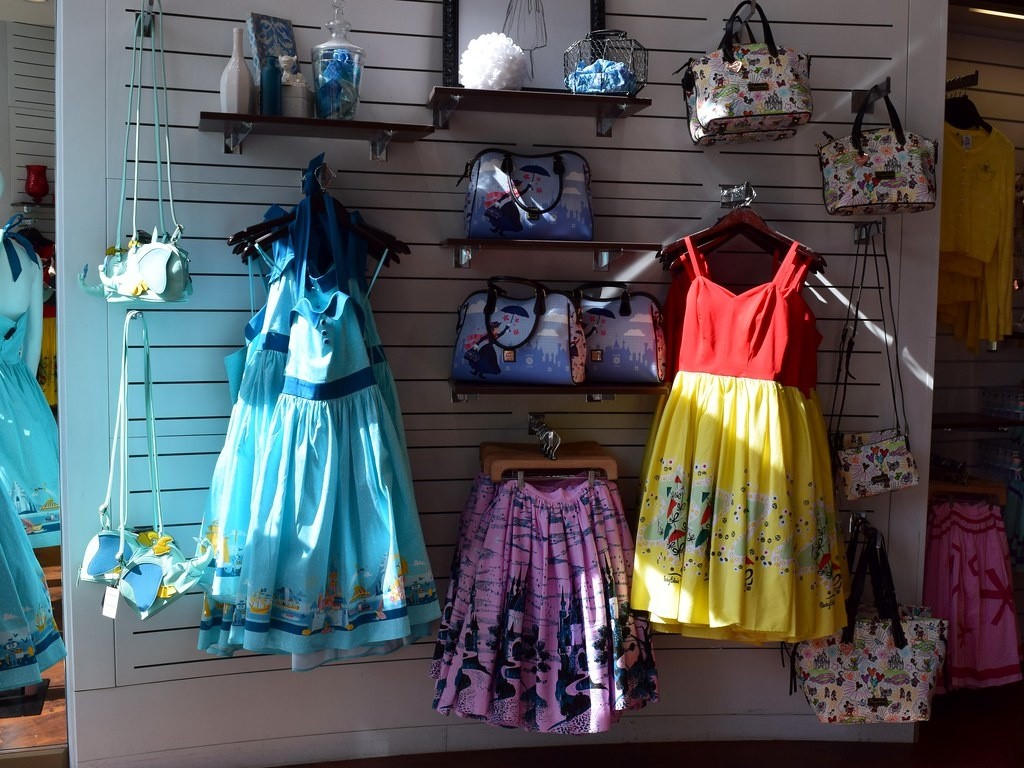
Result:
[202,84,661,269]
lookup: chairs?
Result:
[226,162,412,268]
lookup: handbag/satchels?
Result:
[571,281,665,384]
[563,29,649,95]
[782,510,949,725]
[454,146,593,242]
[670,3,813,147]
[449,276,586,385]
[118,533,214,620]
[837,434,920,501]
[826,427,900,491]
[816,85,938,217]
[76,528,160,585]
[77,234,194,303]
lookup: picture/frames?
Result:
[442,0,606,95]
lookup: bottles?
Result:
[311,0,367,122]
[220,28,256,115]
[260,55,283,116]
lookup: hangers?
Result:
[478,417,618,480]
[945,75,992,134]
[655,185,828,277]
[929,462,1007,505]
